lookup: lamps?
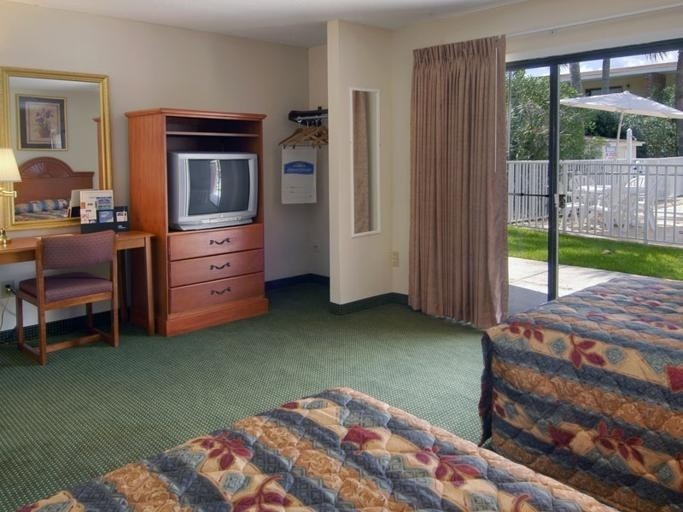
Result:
[0,147,24,245]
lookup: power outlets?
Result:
[0,280,15,298]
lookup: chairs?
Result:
[623,176,655,187]
[620,184,658,231]
[568,175,596,195]
[17,230,119,367]
[557,182,585,231]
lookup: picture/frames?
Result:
[15,93,69,153]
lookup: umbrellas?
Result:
[559,90,682,159]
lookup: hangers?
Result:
[278,117,328,149]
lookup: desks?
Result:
[0,227,156,336]
[579,187,641,234]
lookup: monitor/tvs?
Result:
[168,150,259,231]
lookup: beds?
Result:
[477,276,683,512]
[18,388,617,512]
[12,157,95,222]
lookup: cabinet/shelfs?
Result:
[125,108,268,338]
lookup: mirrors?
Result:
[0,65,114,230]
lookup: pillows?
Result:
[15,204,32,214]
[30,199,68,213]
